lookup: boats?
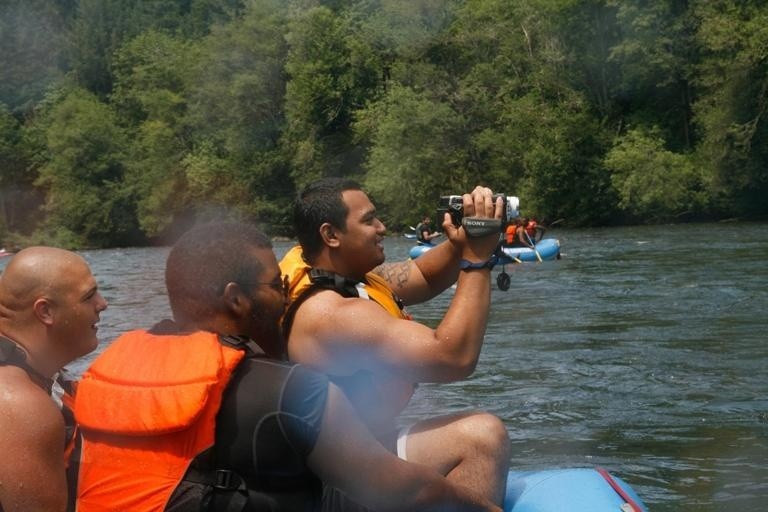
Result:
[495,467,653,512]
[405,237,564,264]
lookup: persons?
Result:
[416,213,547,250]
[276,175,508,510]
[0,244,108,512]
[74,220,502,512]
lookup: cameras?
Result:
[437,193,520,238]
